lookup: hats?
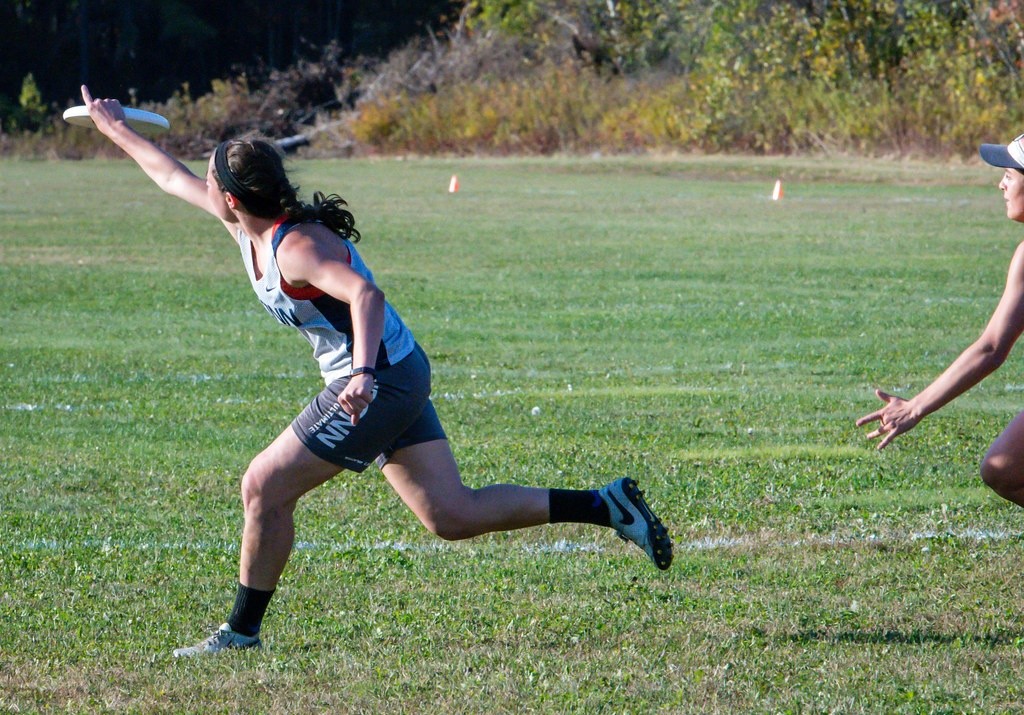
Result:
[978,134,1024,169]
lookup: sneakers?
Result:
[600,476,675,571]
[173,623,265,657]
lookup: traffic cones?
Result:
[769,178,785,202]
[448,174,462,194]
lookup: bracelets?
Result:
[351,367,377,378]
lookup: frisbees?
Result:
[62,103,171,135]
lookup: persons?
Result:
[80,82,675,660]
[853,132,1023,511]
